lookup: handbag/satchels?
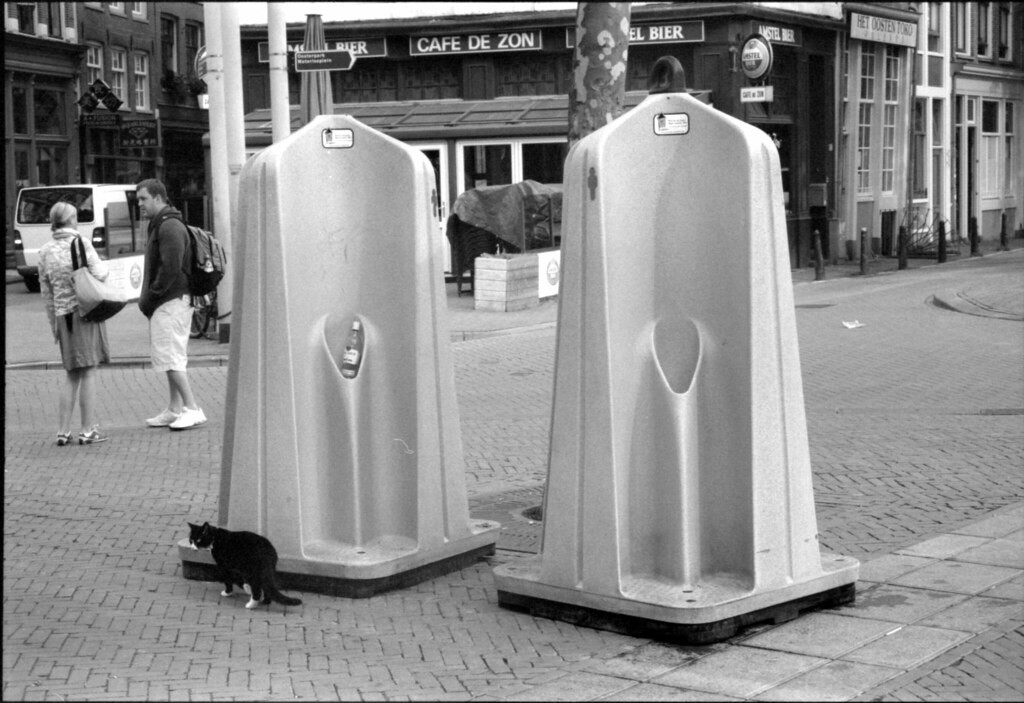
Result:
[67,235,129,323]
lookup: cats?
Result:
[187,521,304,609]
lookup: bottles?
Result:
[203,255,214,273]
[341,321,362,377]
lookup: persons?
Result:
[136,179,206,428]
[39,203,109,444]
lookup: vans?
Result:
[12,182,138,293]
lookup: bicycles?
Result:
[189,288,217,339]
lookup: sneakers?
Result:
[167,407,208,428]
[55,430,74,446]
[78,424,109,445]
[145,408,183,426]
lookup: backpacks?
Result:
[154,214,227,297]
